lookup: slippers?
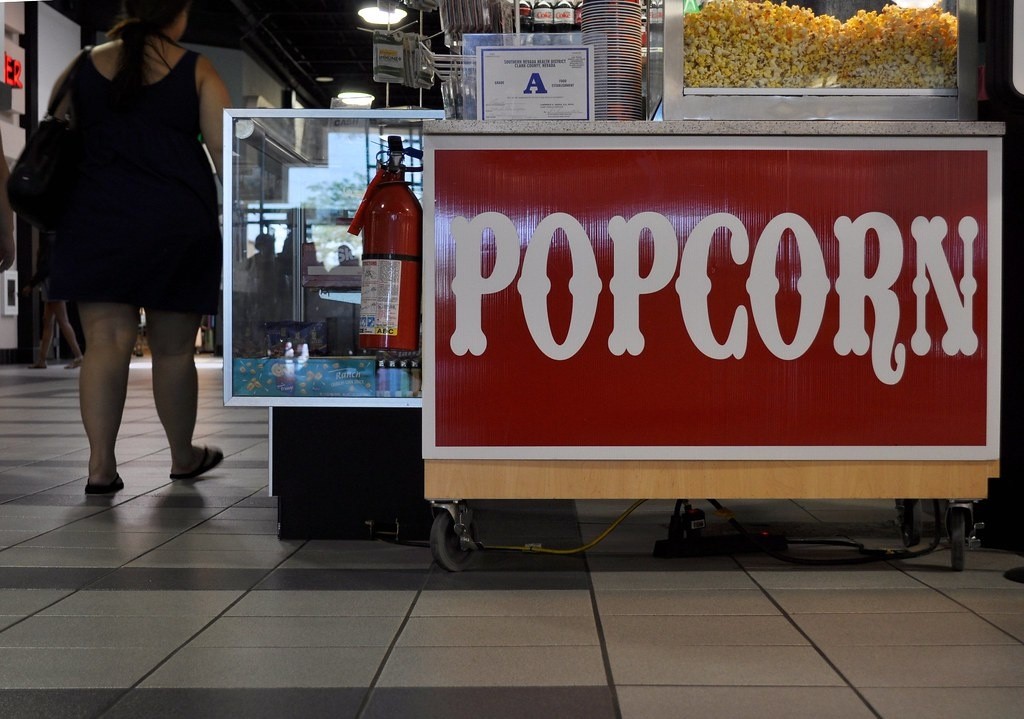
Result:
[84,472,125,496]
[169,446,224,479]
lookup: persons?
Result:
[0,129,16,274]
[42,0,236,496]
[240,203,362,360]
[22,269,84,370]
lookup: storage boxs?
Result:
[646,0,978,121]
[223,108,445,408]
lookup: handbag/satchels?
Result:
[6,45,94,231]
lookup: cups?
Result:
[582,0,641,121]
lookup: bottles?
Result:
[554,0,575,33]
[575,1,582,33]
[512,0,533,33]
[534,0,554,33]
[650,0,664,47]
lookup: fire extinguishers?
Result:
[347,135,424,351]
